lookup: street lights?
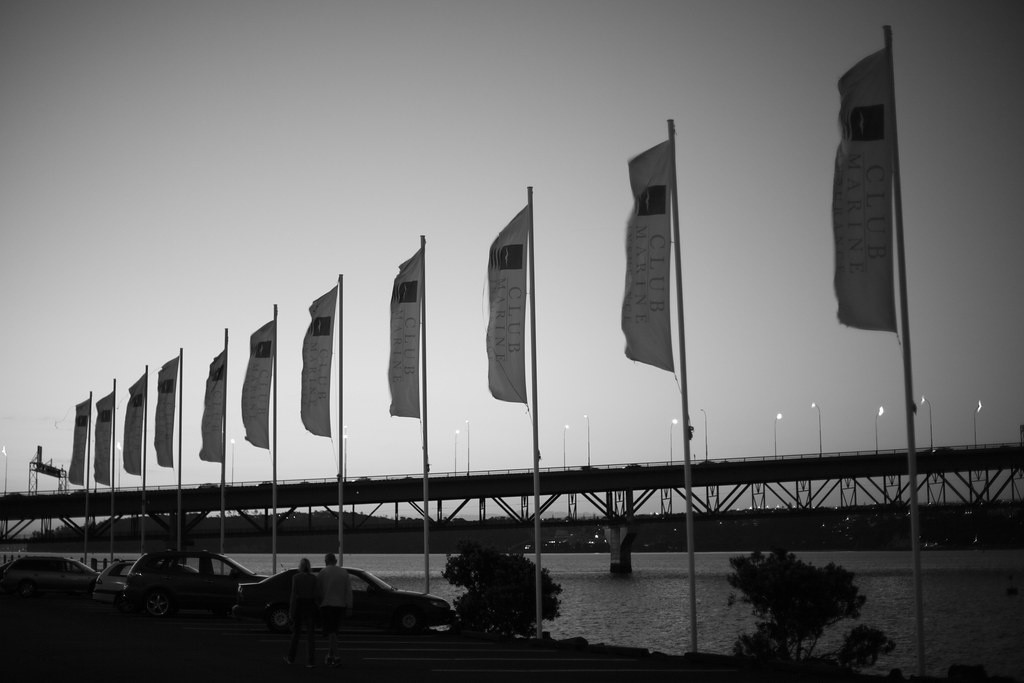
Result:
[455,430,460,477]
[465,419,470,478]
[774,414,782,460]
[700,409,708,461]
[875,407,884,454]
[811,402,822,458]
[583,415,591,466]
[921,398,933,452]
[564,425,570,471]
[343,425,349,483]
[230,439,236,487]
[670,419,678,466]
[973,405,983,449]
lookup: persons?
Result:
[280,558,320,667]
[317,554,352,667]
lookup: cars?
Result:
[236,567,452,636]
[91,558,200,615]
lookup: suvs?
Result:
[0,555,103,601]
[122,549,274,621]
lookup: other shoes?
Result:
[334,657,343,667]
[304,664,316,668]
[282,656,295,665]
[325,655,333,666]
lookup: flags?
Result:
[200,349,225,463]
[388,247,422,417]
[301,284,338,438]
[154,357,179,468]
[487,206,529,403]
[242,319,275,449]
[69,399,91,485]
[94,391,114,485]
[123,374,146,476]
[621,140,675,373]
[833,49,896,333]
